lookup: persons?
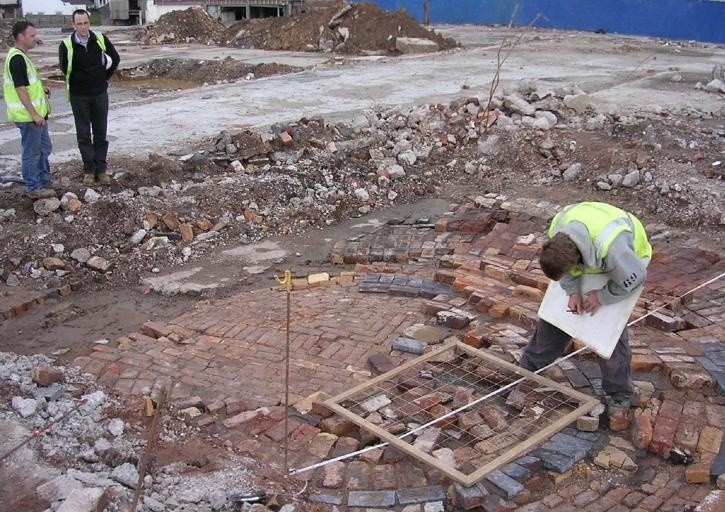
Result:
[2,21,58,200]
[498,200,654,420]
[58,9,120,188]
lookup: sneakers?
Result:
[606,398,631,418]
[83,173,97,187]
[98,173,111,185]
[27,187,56,198]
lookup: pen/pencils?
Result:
[566,310,576,312]
